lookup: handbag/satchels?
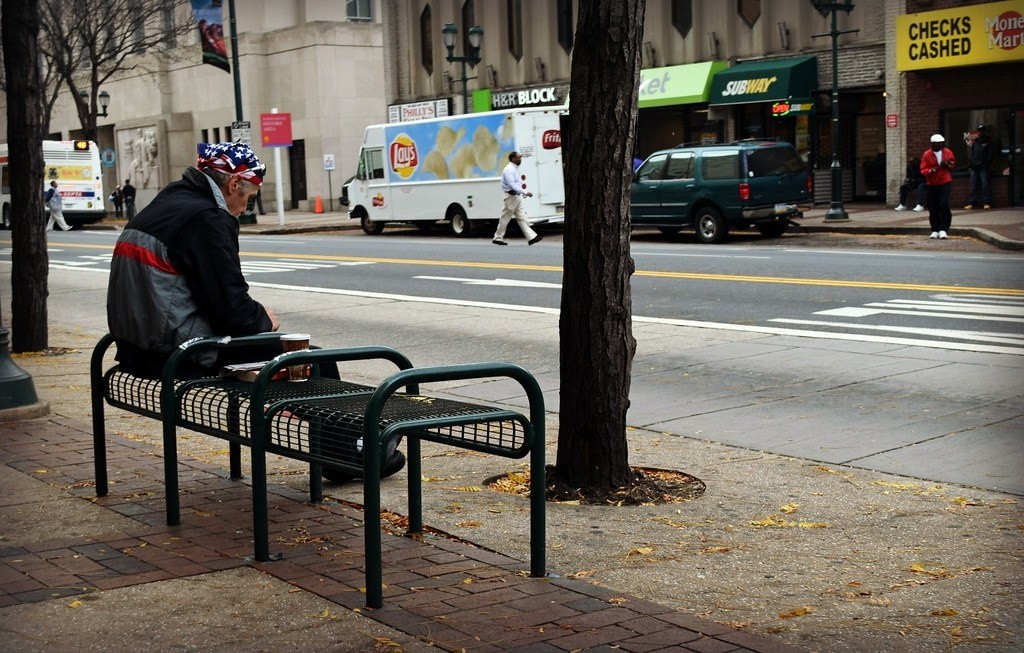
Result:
[109,195,115,201]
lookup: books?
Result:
[238,368,310,382]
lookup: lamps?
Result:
[484,21,790,91]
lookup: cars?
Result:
[339,174,356,205]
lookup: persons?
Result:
[106,140,407,482]
[44,181,73,231]
[120,179,136,220]
[493,152,544,245]
[894,157,928,211]
[111,185,124,218]
[920,134,956,239]
[963,125,994,209]
[251,189,265,215]
[633,146,654,172]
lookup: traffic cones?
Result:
[314,195,324,213]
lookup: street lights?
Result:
[441,22,484,114]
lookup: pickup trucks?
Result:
[626,137,814,244]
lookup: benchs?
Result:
[90,332,545,608]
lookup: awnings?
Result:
[707,56,817,106]
[637,60,729,107]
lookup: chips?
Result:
[421,115,513,180]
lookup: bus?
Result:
[0,140,108,230]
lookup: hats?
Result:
[976,125,988,132]
[930,133,944,143]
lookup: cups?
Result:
[279,333,311,382]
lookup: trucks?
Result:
[347,105,571,238]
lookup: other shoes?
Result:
[528,236,543,244]
[326,449,405,483]
[67,226,73,231]
[984,204,990,210]
[964,204,973,209]
[492,240,508,246]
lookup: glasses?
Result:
[231,164,266,178]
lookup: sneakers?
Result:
[929,230,938,239]
[938,230,949,239]
[912,204,925,212]
[895,204,907,212]
